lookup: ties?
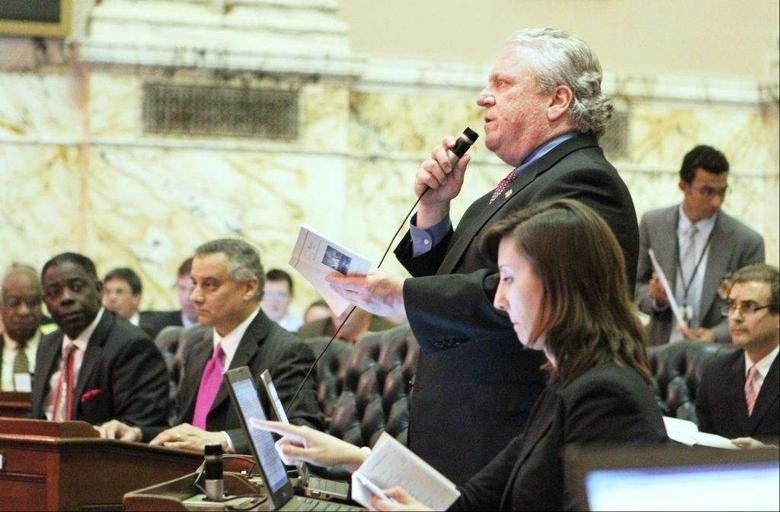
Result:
[190,339,226,432]
[49,342,76,424]
[674,225,701,324]
[743,365,759,417]
[487,167,519,207]
[11,342,31,393]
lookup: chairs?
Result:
[329,321,422,449]
[653,338,735,429]
[301,332,358,434]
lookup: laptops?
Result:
[221,364,366,512]
[260,367,350,501]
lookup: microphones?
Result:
[419,125,480,196]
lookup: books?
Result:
[282,223,374,321]
[348,432,464,512]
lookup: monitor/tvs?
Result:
[559,443,780,512]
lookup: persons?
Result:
[101,259,198,338]
[324,30,641,511]
[261,269,336,330]
[692,264,778,451]
[0,264,54,391]
[247,193,671,510]
[94,239,320,452]
[30,253,169,428]
[631,143,769,348]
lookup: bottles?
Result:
[204,443,229,500]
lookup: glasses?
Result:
[718,299,774,318]
[690,182,732,198]
